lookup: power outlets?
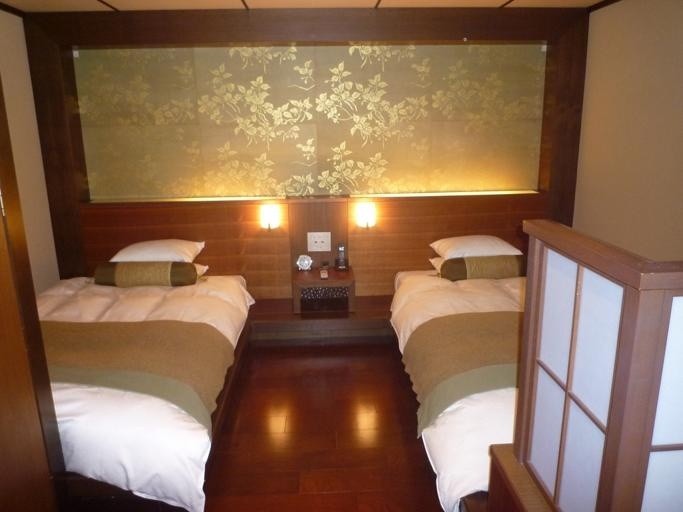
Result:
[307,232,331,251]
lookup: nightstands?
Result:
[293,266,355,314]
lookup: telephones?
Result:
[338,241,345,267]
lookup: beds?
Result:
[37,277,255,512]
[389,270,528,512]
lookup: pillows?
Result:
[110,239,205,263]
[193,263,209,280]
[429,257,444,274]
[440,255,526,280]
[429,235,524,258]
[95,262,197,288]
